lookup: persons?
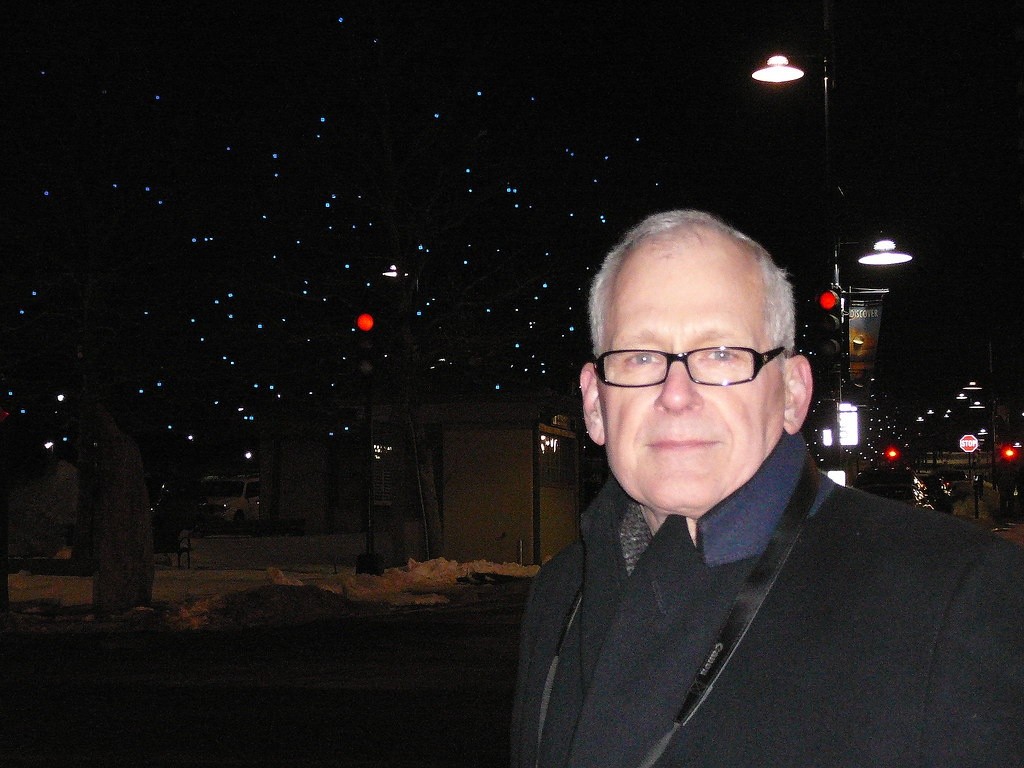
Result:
[512,211,1024,768]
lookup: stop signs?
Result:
[959,434,978,453]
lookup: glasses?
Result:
[594,346,787,387]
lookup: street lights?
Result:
[753,49,838,286]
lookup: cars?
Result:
[852,465,953,514]
